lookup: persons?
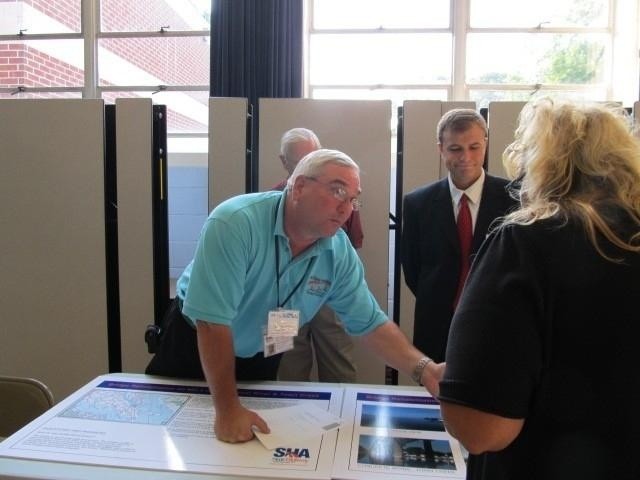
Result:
[401,109,521,364]
[437,98,640,480]
[270,128,364,384]
[145,149,447,443]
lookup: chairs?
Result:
[0,376,55,437]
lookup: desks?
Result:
[0,373,470,480]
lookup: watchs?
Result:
[413,356,432,385]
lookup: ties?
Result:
[453,193,474,313]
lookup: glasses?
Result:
[504,172,526,201]
[305,176,362,212]
